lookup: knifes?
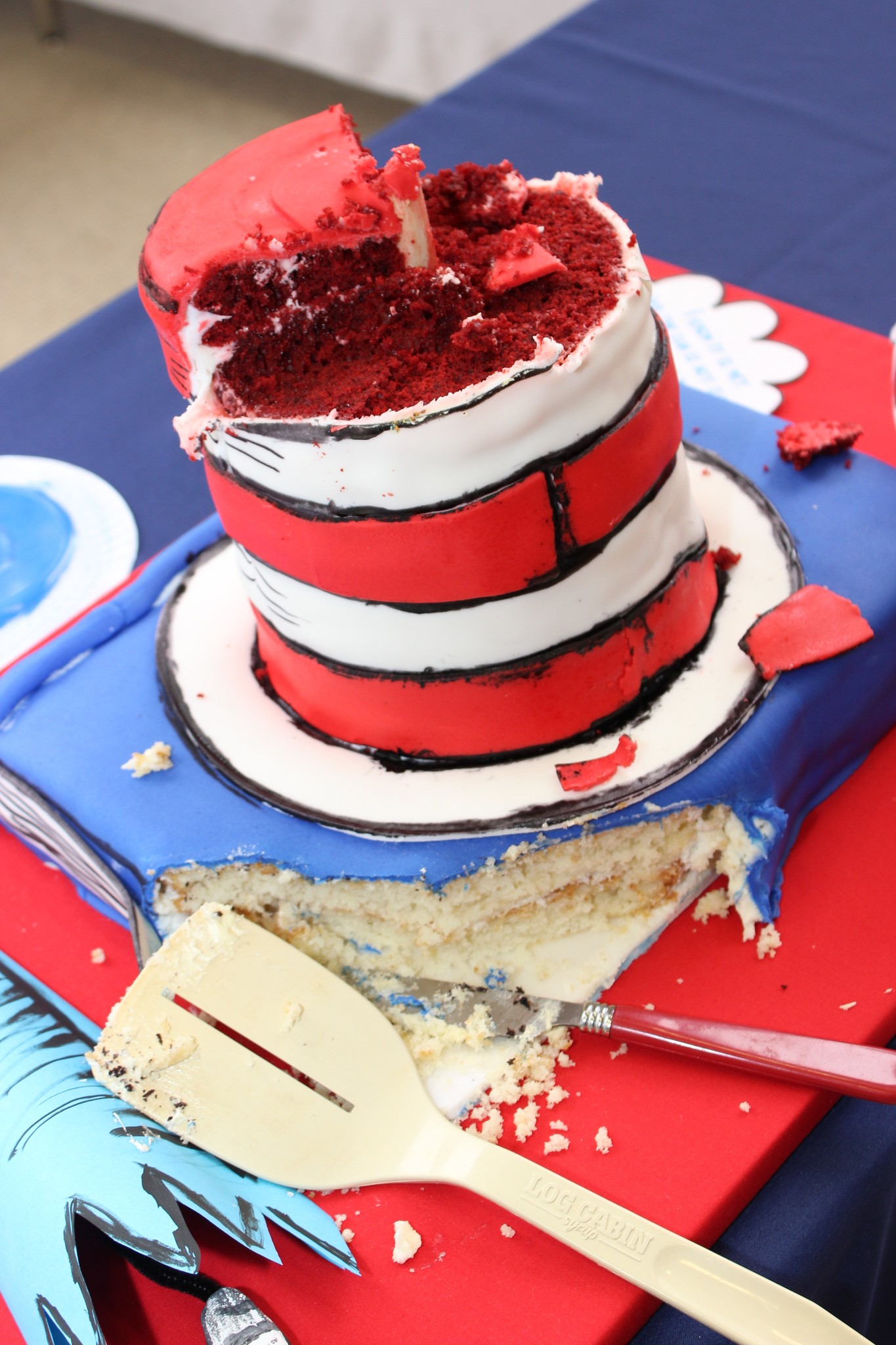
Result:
[334,965,896,1104]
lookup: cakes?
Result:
[0,103,896,1125]
